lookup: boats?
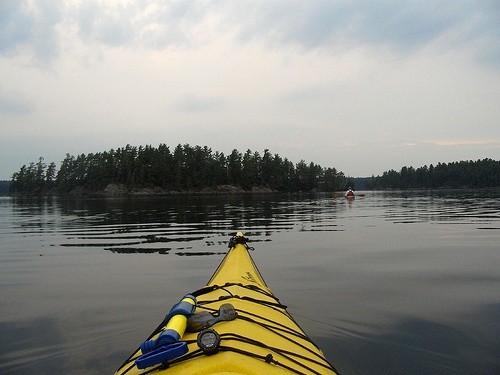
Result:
[346,191,355,196]
[110,231,344,374]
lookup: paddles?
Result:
[355,194,365,197]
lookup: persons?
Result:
[344,186,355,197]
